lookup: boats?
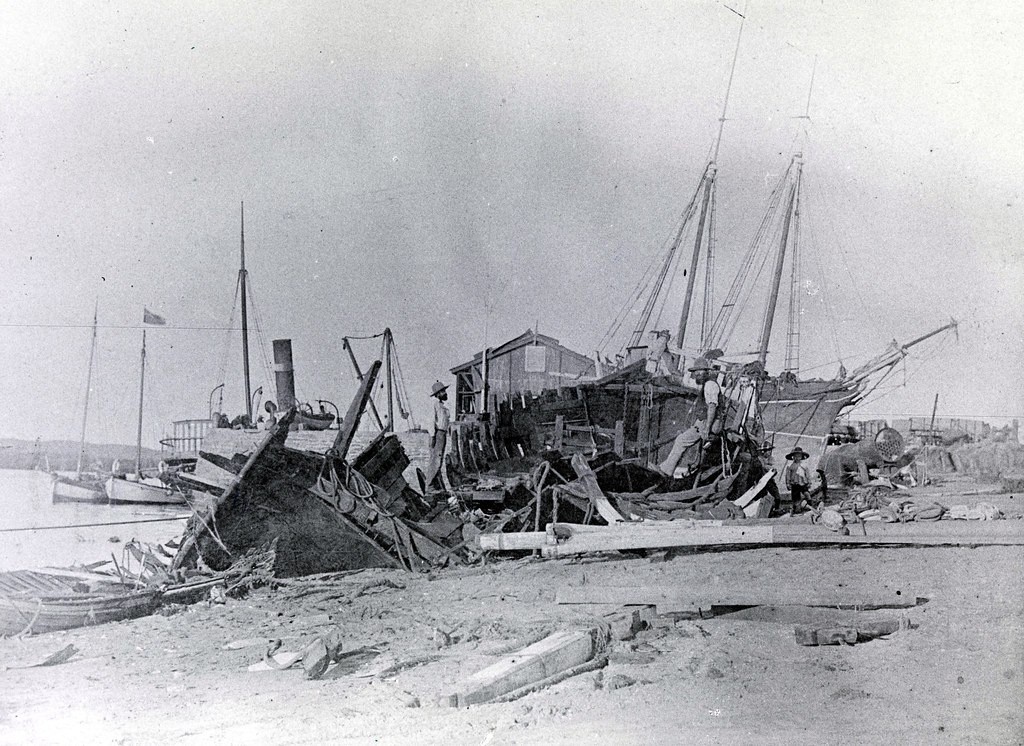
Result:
[159,1,961,518]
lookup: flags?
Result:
[143,309,166,325]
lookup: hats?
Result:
[429,381,450,397]
[758,441,774,452]
[785,447,810,460]
[688,358,715,372]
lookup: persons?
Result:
[648,358,725,477]
[427,382,450,454]
[785,446,810,512]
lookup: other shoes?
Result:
[649,461,669,478]
[429,489,445,494]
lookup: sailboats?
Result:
[35,289,106,503]
[106,305,190,504]
